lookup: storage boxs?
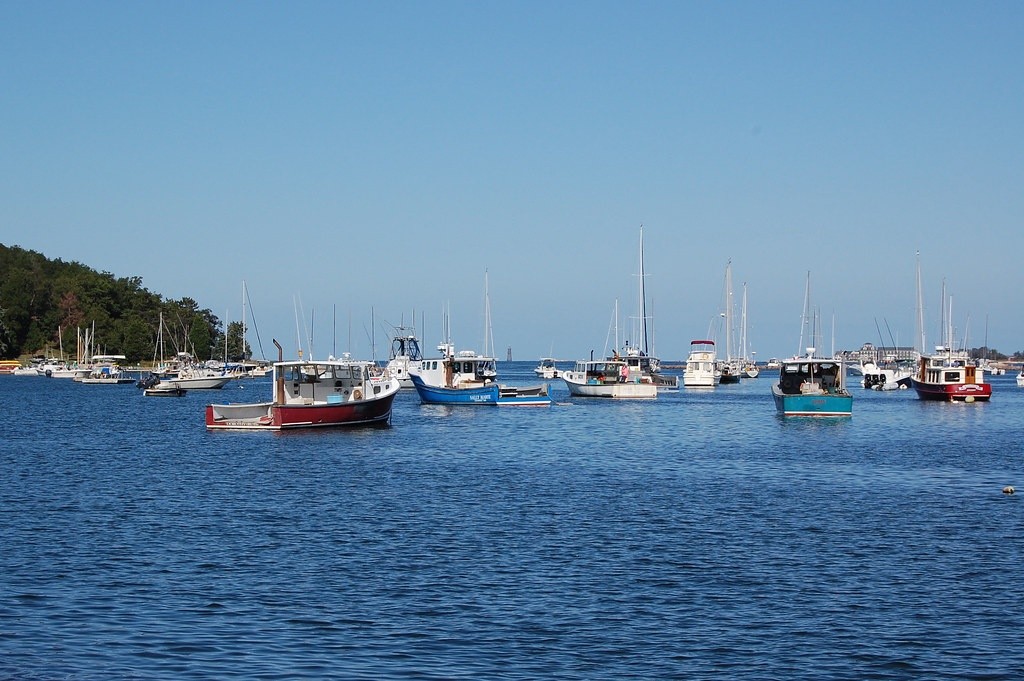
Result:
[327,394,344,404]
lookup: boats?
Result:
[0,204,1024,439]
